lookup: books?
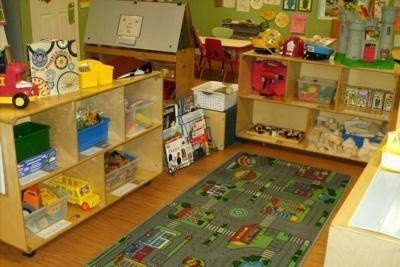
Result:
[161,94,208,174]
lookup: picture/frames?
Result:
[283,0,296,11]
[298,0,312,12]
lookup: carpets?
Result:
[79,151,353,267]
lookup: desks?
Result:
[322,132,400,266]
[195,36,253,59]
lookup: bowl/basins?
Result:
[212,27,234,38]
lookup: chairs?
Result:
[199,38,235,82]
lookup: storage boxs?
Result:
[204,106,236,151]
[105,150,139,193]
[297,76,339,104]
[24,185,71,232]
[124,96,155,135]
[14,119,52,162]
[79,114,112,152]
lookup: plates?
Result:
[250,0,263,9]
[275,13,289,28]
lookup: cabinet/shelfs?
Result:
[85,46,196,101]
[19,0,80,71]
[0,56,164,256]
[235,49,400,164]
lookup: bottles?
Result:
[363,43,376,62]
